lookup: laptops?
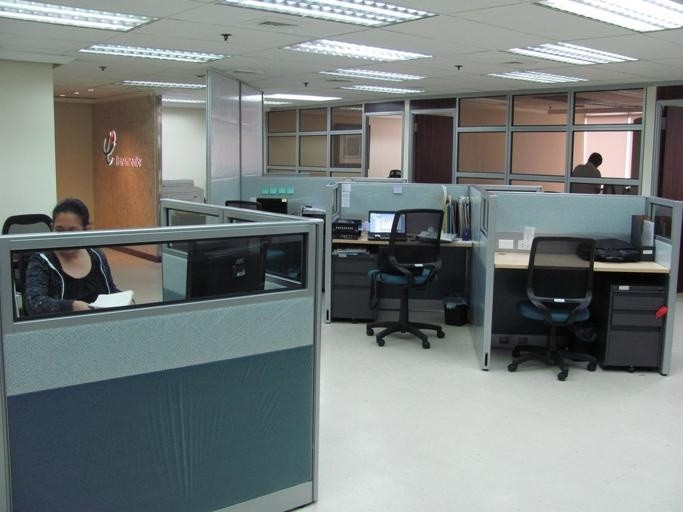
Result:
[368,210,407,241]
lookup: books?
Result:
[442,184,470,238]
[331,244,372,256]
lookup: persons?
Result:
[22,198,135,317]
[571,152,603,193]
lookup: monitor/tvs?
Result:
[186,237,266,297]
[257,197,287,214]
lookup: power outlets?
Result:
[517,240,531,251]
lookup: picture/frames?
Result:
[333,123,370,171]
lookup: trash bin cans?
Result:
[569,326,603,362]
[442,297,469,326]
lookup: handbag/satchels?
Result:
[576,238,641,262]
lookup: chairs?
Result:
[505,234,600,381]
[219,197,286,273]
[1,211,56,316]
[355,207,448,351]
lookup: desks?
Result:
[331,236,473,324]
[492,248,669,375]
